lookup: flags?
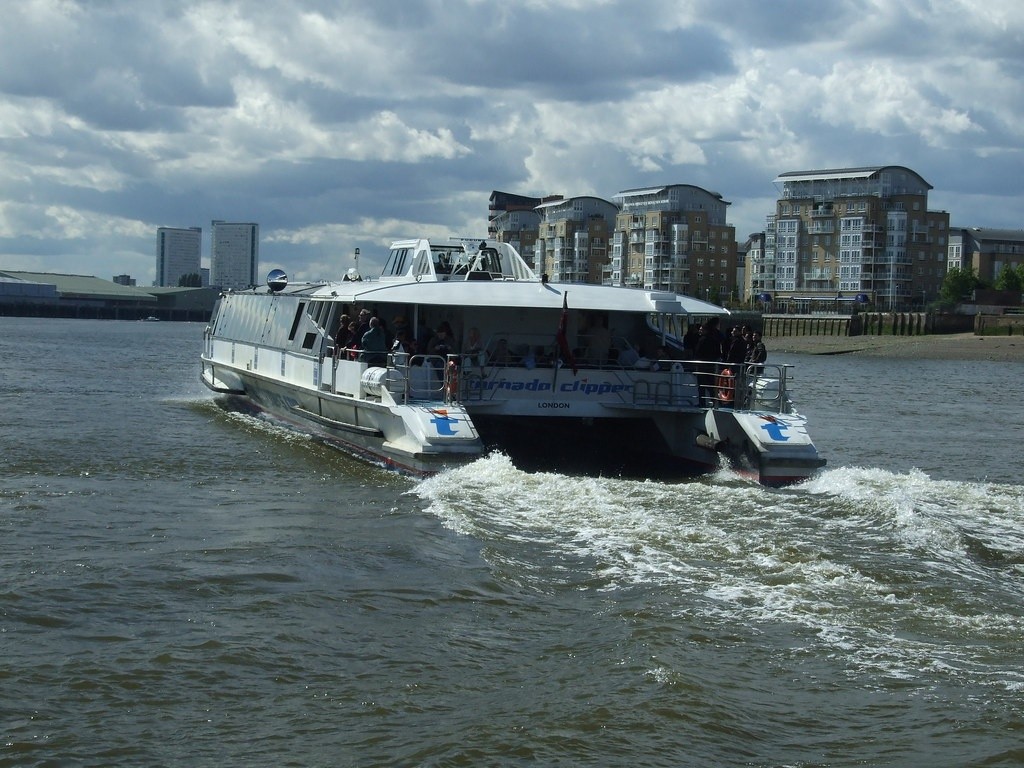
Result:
[552,302,570,359]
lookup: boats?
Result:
[199,234,830,488]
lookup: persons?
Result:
[435,253,452,274]
[334,309,767,408]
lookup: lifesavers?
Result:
[719,370,735,403]
[443,362,460,391]
